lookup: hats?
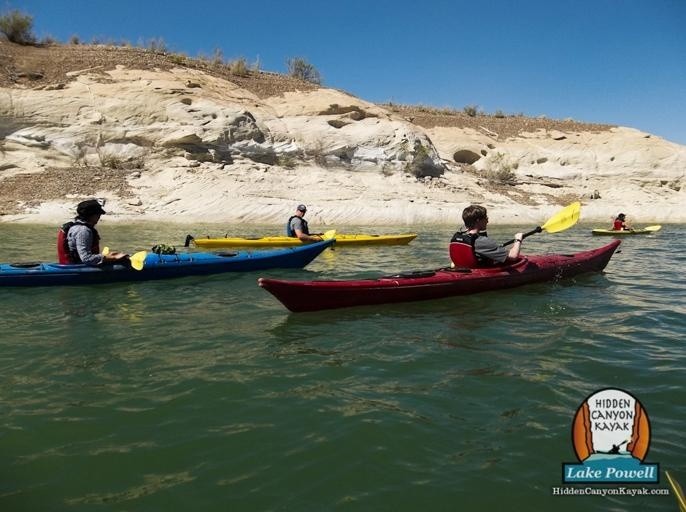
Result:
[297,204,306,211]
[75,199,107,219]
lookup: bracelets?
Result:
[512,239,521,243]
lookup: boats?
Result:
[592,225,662,234]
[258,240,621,312]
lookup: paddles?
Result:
[482,201,580,253]
[624,225,662,231]
[309,229,336,239]
[102,246,147,271]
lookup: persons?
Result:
[447,204,524,270]
[612,213,634,230]
[286,203,323,243]
[59,200,128,267]
